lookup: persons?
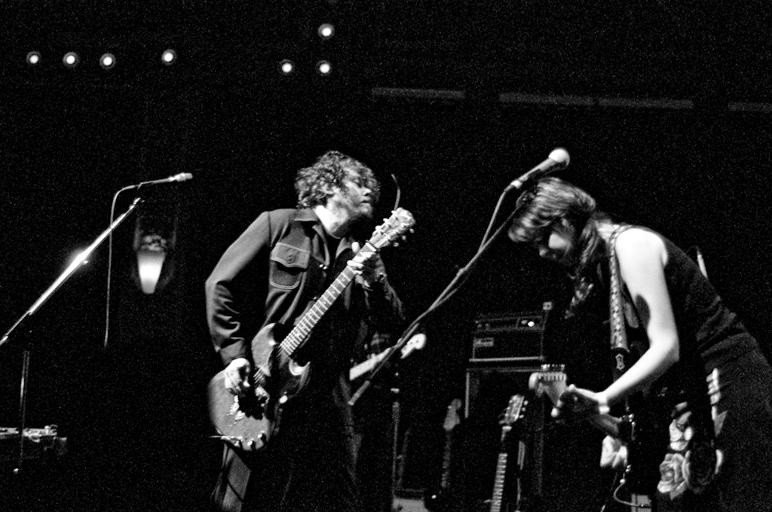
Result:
[508,175,771,512]
[205,151,405,511]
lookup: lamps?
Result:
[135,233,166,297]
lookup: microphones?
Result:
[122,172,193,191]
[506,148,570,195]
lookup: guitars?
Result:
[490,395,531,511]
[528,363,657,508]
[207,207,416,451]
